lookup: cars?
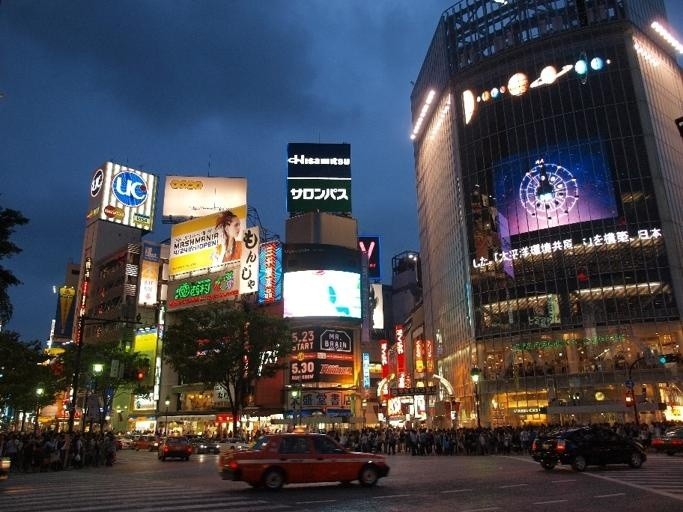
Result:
[219,432,390,492]
[531,427,646,471]
[116,434,220,461]
[652,426,683,456]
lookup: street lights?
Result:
[89,357,104,432]
[471,368,482,426]
[33,381,45,435]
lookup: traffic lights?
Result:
[659,356,667,364]
[136,371,145,380]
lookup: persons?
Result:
[215,210,241,263]
[0,430,114,473]
